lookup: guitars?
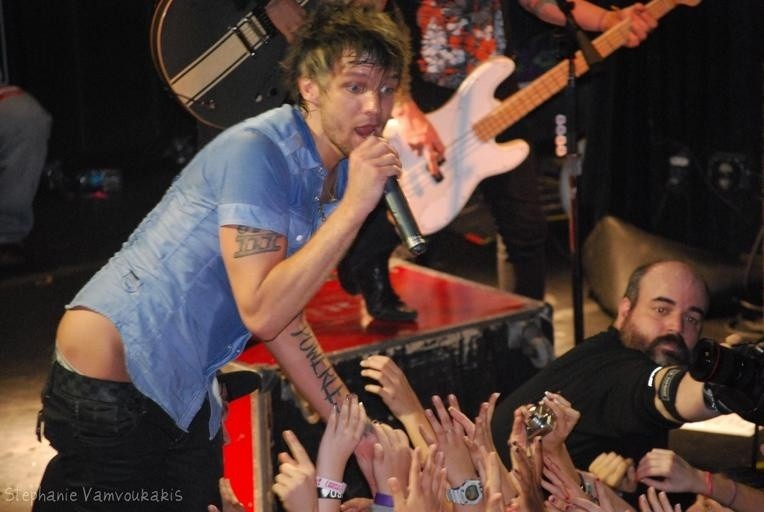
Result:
[149,0,311,130]
[379,1,700,237]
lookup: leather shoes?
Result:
[338,263,417,322]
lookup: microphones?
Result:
[383,176,429,255]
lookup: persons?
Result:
[0,64,52,267]
[269,261,764,512]
[260,0,419,326]
[25,26,405,512]
[344,1,659,302]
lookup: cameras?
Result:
[526,414,552,439]
[687,337,764,434]
